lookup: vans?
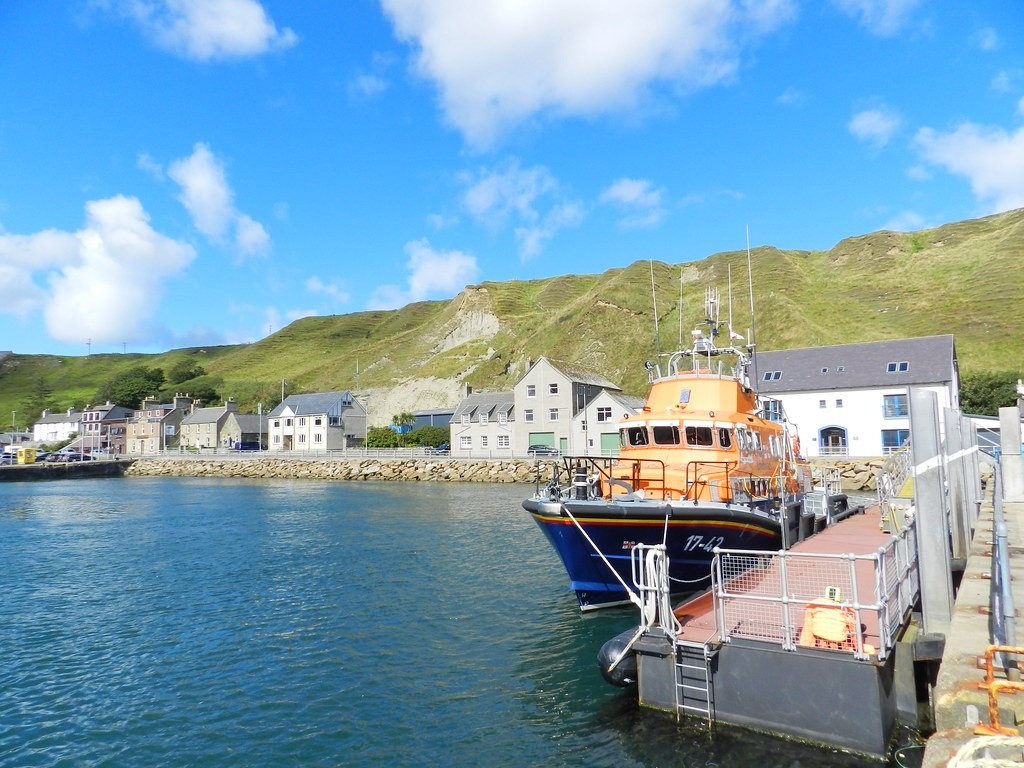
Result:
[235,442,259,452]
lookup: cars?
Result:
[526,444,562,457]
[0,454,18,466]
[46,452,62,462]
[436,444,450,455]
[62,452,96,462]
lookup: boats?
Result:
[519,222,811,615]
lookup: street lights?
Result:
[10,410,17,466]
